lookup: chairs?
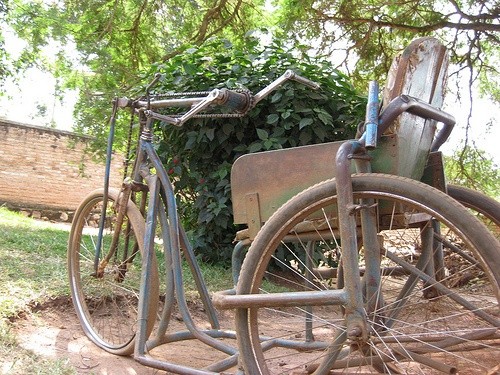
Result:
[228,36,458,354]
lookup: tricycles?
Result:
[68,33,500,375]
